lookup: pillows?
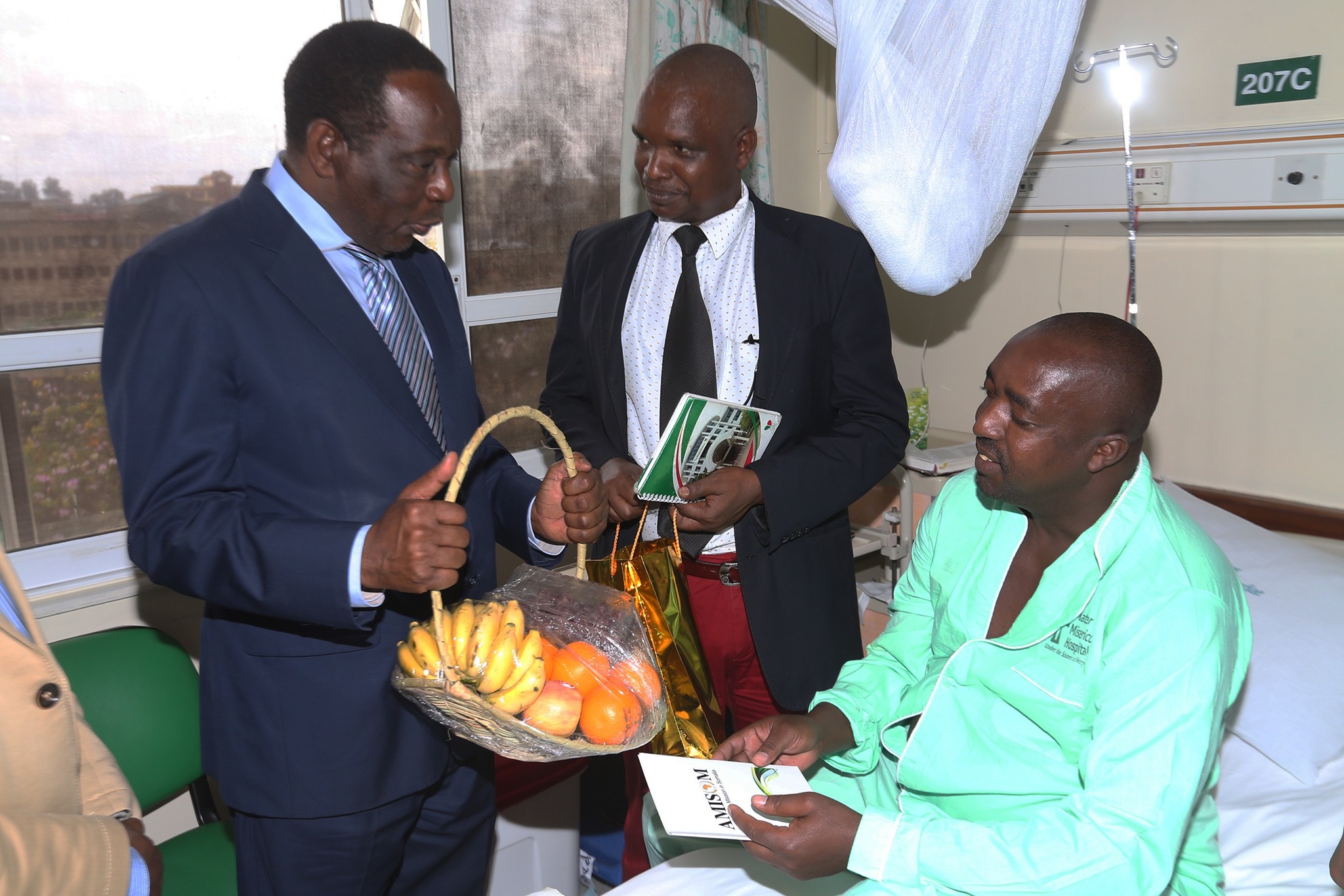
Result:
[1216,733,1343,807]
[1149,483,1343,786]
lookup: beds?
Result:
[601,474,1344,896]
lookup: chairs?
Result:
[50,624,236,896]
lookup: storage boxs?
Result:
[484,752,589,896]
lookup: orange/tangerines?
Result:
[527,632,662,745]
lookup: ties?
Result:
[658,225,720,560]
[346,242,446,458]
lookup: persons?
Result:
[1,550,163,896]
[539,45,911,890]
[641,312,1254,896]
[100,20,612,895]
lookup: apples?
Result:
[520,679,582,739]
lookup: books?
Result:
[633,392,782,505]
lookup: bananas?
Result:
[396,599,546,716]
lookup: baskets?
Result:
[393,406,667,763]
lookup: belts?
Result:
[682,558,742,588]
